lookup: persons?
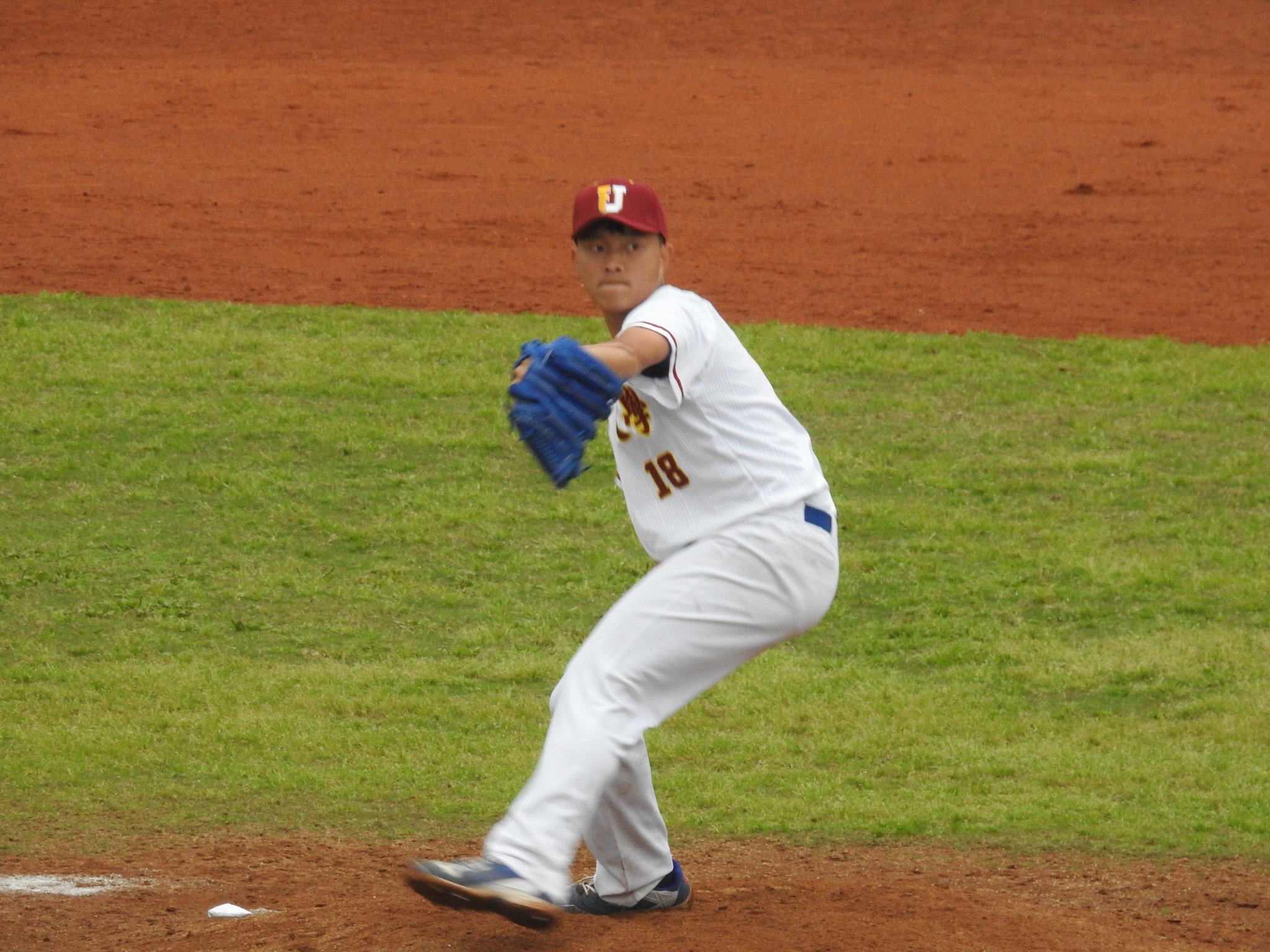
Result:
[402,178,841,929]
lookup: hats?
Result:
[569,179,667,244]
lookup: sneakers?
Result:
[568,859,691,913]
[404,854,556,930]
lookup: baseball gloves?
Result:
[505,333,626,491]
